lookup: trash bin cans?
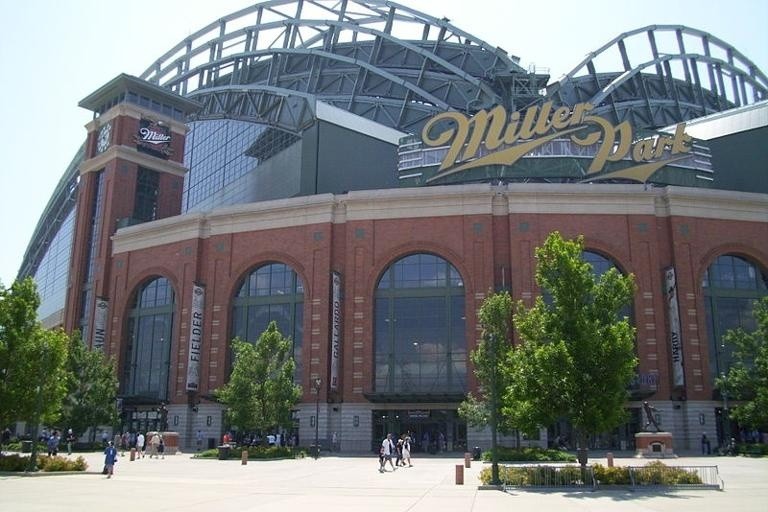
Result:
[218,446,230,460]
[473,447,481,461]
[22,441,33,452]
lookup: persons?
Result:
[195,430,207,451]
[104,441,117,478]
[223,431,297,447]
[438,431,446,455]
[727,437,736,455]
[157,402,169,431]
[377,432,413,474]
[2,427,75,456]
[701,432,711,455]
[102,430,167,460]
[332,431,338,451]
[422,431,430,452]
[643,401,665,432]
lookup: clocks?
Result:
[95,123,113,153]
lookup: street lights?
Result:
[311,376,323,460]
